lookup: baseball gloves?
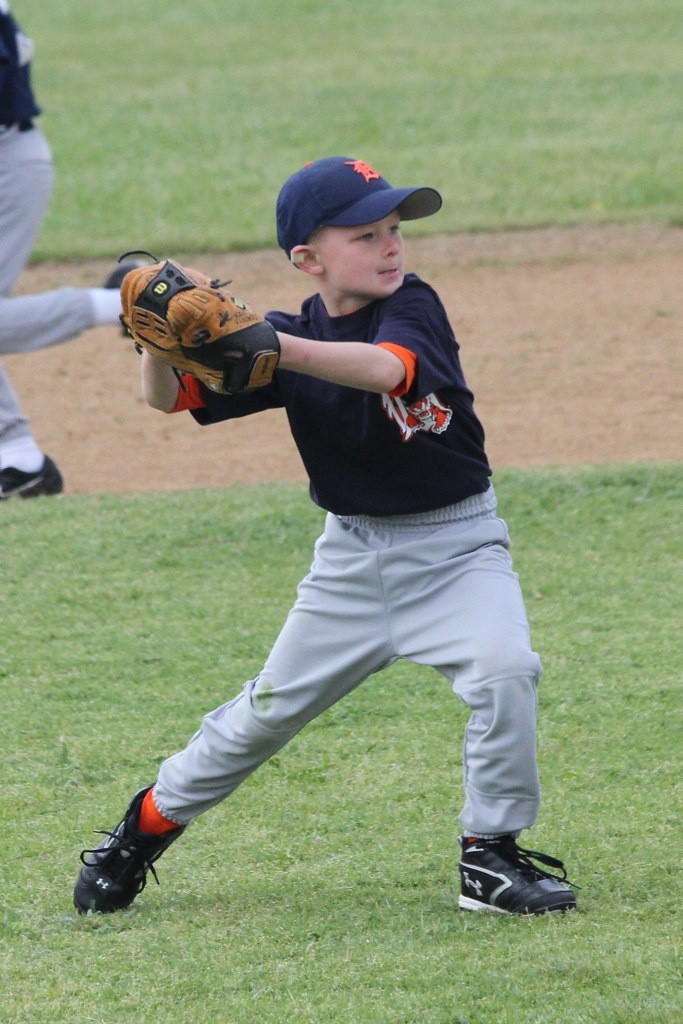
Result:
[119,258,282,397]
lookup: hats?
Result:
[275,157,443,251]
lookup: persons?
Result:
[0,0,151,500]
[72,155,577,915]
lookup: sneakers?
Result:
[0,454,63,500]
[457,833,581,916]
[73,781,188,915]
